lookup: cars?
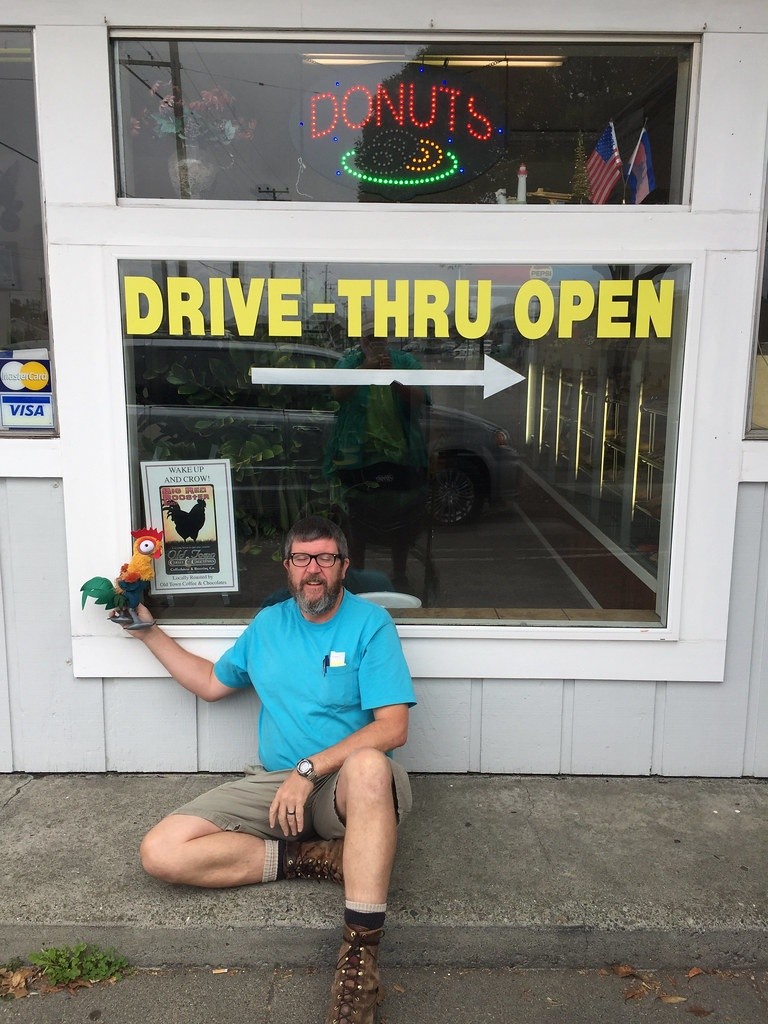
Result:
[401,337,511,359]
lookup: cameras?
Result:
[368,340,388,358]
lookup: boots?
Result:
[283,840,344,889]
[324,920,385,1024]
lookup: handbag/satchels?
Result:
[344,489,425,546]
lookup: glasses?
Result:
[286,551,344,568]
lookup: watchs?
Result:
[297,758,319,783]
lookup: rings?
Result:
[287,812,297,816]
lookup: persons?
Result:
[109,515,418,1024]
[334,315,432,581]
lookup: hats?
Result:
[361,310,374,336]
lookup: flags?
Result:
[630,130,656,204]
[582,123,624,204]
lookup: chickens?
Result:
[160,497,206,542]
[79,528,165,628]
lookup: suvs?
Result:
[124,333,520,528]
